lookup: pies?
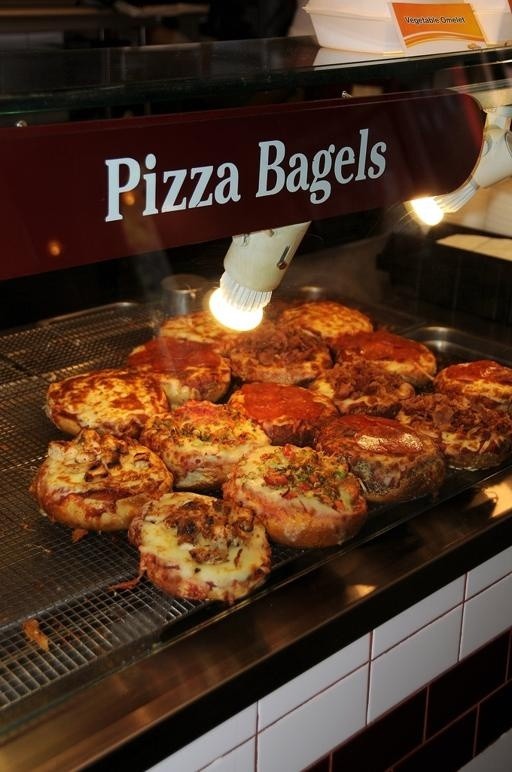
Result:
[27,297,512,602]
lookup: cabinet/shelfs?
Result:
[0,29,512,330]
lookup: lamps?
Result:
[402,104,511,227]
[209,222,310,332]
